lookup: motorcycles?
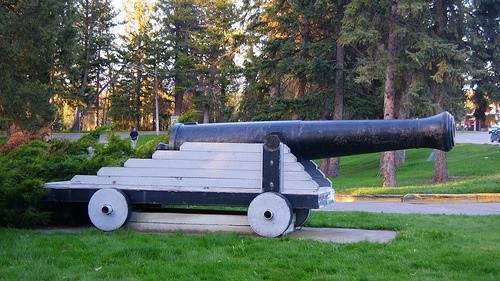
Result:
[489,126,500,143]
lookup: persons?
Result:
[93,126,100,139]
[130,127,138,148]
